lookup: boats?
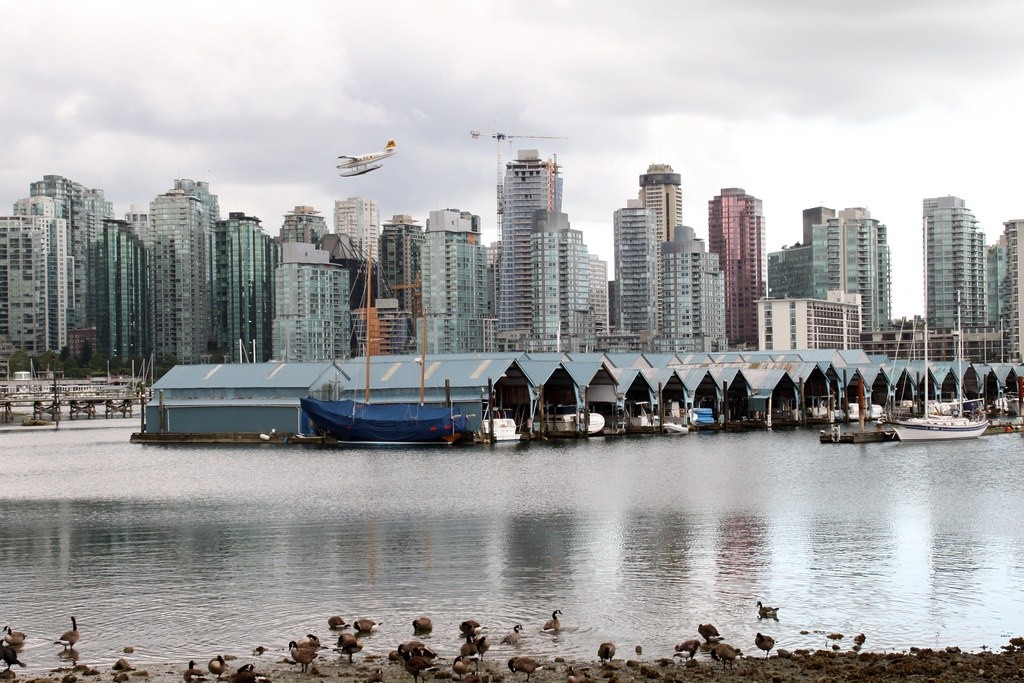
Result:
[473,388,922,443]
[880,291,990,443]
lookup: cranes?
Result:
[469,129,570,240]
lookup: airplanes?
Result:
[336,140,398,177]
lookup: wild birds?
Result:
[53,616,80,650]
[229,664,271,683]
[541,609,563,632]
[413,618,433,635]
[389,642,448,683]
[353,618,383,633]
[328,616,351,629]
[598,643,616,664]
[0,639,27,669]
[297,634,329,652]
[289,640,318,672]
[499,624,524,643]
[453,620,490,681]
[2,625,27,644]
[672,601,779,669]
[332,634,363,664]
[56,649,79,666]
[184,660,206,683]
[209,656,227,682]
[508,656,544,683]
